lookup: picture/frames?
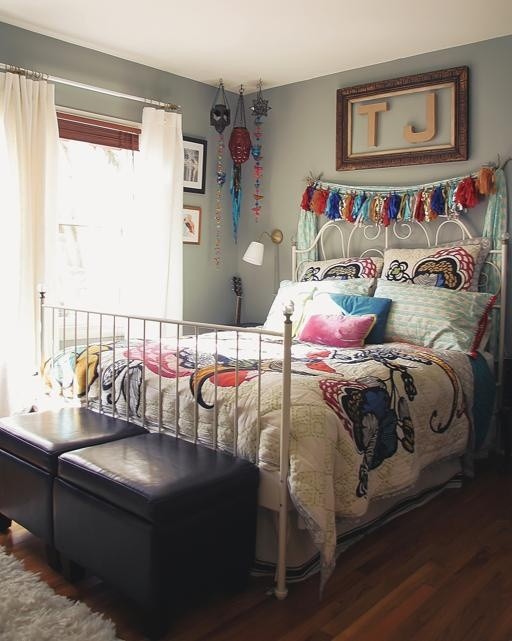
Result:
[333,64,470,172]
[182,136,208,245]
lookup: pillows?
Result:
[261,235,500,363]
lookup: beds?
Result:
[38,217,507,603]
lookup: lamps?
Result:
[239,229,283,267]
